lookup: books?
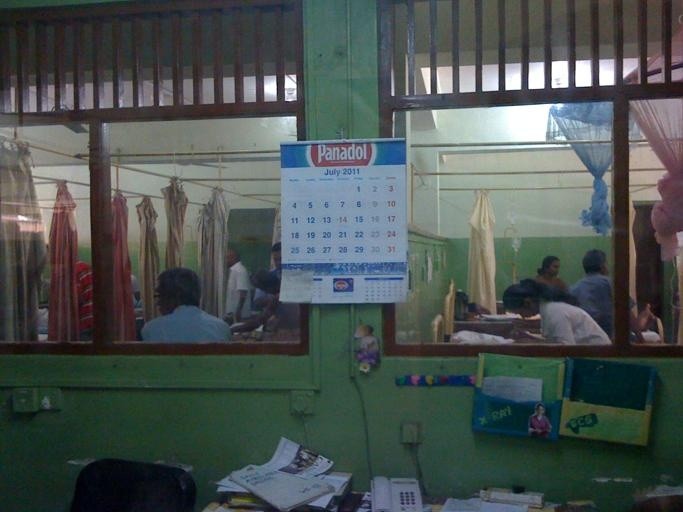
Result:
[212,434,354,512]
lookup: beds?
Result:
[431,282,544,344]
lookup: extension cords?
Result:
[479,487,545,509]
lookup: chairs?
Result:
[70,456,197,511]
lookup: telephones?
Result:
[371,475,423,512]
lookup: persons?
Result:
[500,279,610,343]
[531,257,571,292]
[566,251,645,344]
[73,241,300,341]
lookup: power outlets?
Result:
[291,389,315,415]
